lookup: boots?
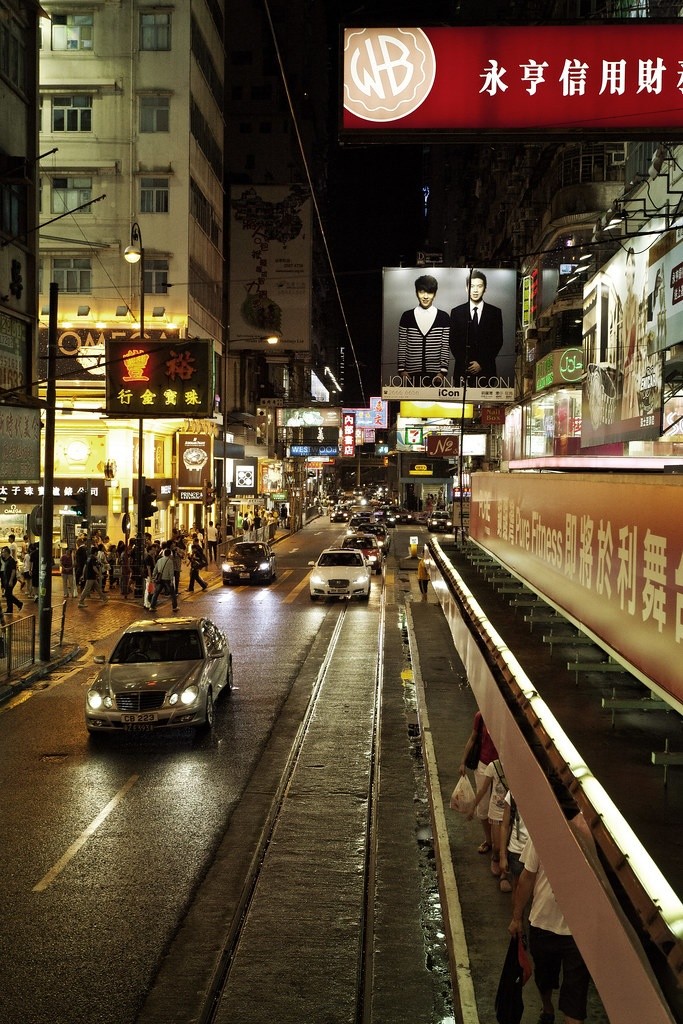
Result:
[421,593,427,603]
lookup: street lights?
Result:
[220,335,279,553]
[125,223,150,541]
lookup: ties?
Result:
[472,307,479,329]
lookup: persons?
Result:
[397,275,450,387]
[60,534,208,611]
[449,272,503,387]
[621,247,639,420]
[172,521,218,562]
[237,504,287,534]
[460,711,589,1023]
[0,534,39,633]
[287,410,300,426]
[418,549,430,602]
[425,493,436,512]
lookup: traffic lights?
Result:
[71,493,88,518]
[203,487,216,505]
[143,485,158,528]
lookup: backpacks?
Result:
[243,518,249,531]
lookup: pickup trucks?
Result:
[427,510,454,534]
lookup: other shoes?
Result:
[173,607,180,610]
[478,840,555,1024]
[63,593,69,598]
[149,608,158,612]
[25,591,30,595]
[78,603,88,607]
[4,610,13,614]
[28,595,34,598]
[184,588,194,592]
[201,582,208,590]
[18,602,23,611]
[104,596,111,603]
[2,595,5,598]
[33,595,39,602]
[20,582,26,590]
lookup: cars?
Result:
[325,488,358,522]
[220,541,276,584]
[355,523,392,555]
[308,547,377,602]
[350,483,413,529]
[342,531,381,575]
[83,617,232,736]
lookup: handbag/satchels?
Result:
[191,555,208,570]
[494,933,531,1024]
[450,773,476,813]
[155,551,164,561]
[148,580,155,594]
[153,572,162,583]
[102,563,111,572]
[465,716,483,770]
